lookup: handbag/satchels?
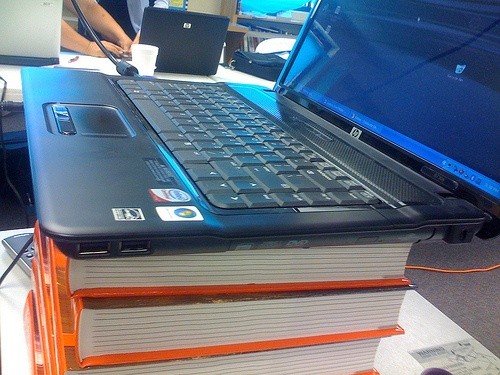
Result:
[231,51,290,82]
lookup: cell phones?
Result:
[3,233,34,279]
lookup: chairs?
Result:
[0,113,32,229]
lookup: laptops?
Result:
[0,0,62,67]
[22,0,500,256]
[138,6,230,76]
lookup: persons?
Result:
[78,0,170,51]
[60,0,133,58]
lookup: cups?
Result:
[131,43,159,76]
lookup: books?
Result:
[37,243,418,375]
